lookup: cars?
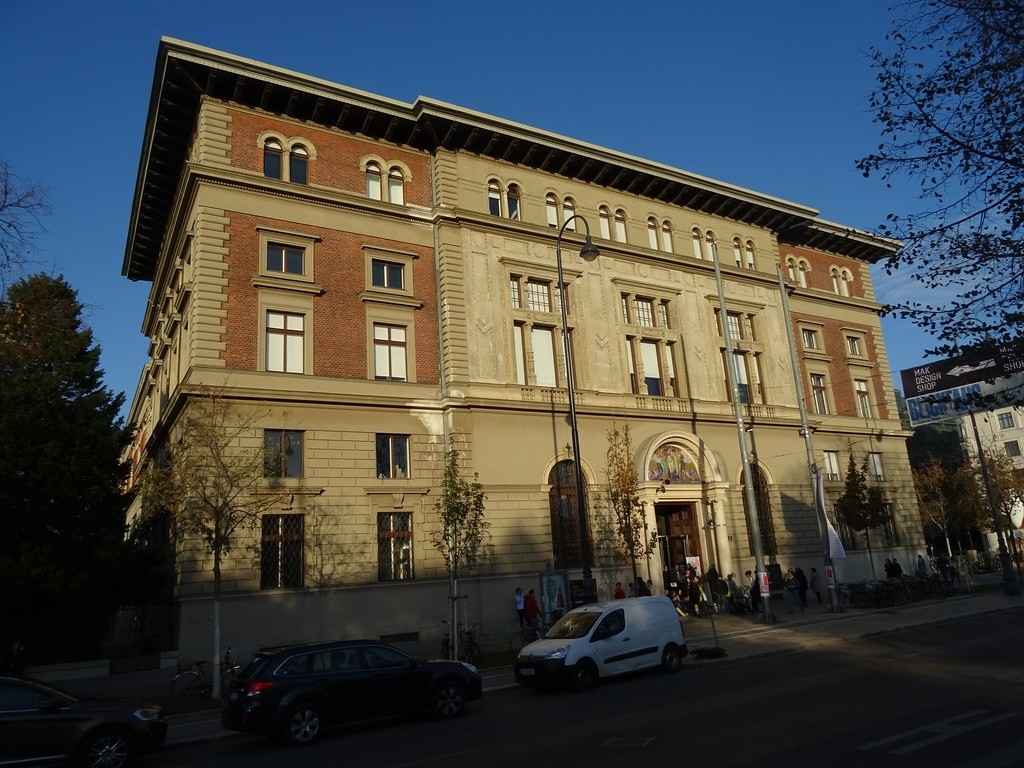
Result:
[219,639,486,748]
[0,671,171,768]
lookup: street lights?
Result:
[553,213,602,603]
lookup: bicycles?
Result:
[169,645,242,709]
[727,583,766,618]
[835,571,957,607]
[438,609,561,665]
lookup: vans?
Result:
[512,595,690,691]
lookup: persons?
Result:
[629,577,655,597]
[663,563,824,615]
[613,582,625,599]
[885,555,961,588]
[514,588,543,628]
